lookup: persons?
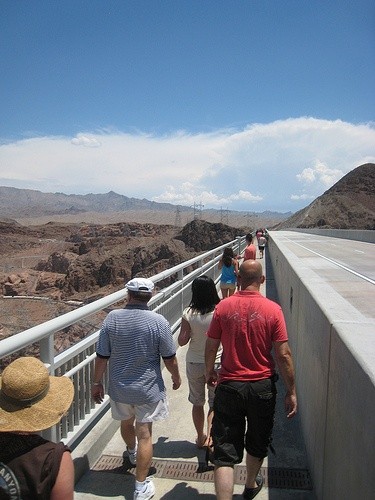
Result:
[256,228,269,259]
[218,247,239,299]
[242,234,257,263]
[0,356,75,500]
[205,259,298,500]
[178,275,225,447]
[91,278,180,500]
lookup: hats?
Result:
[125,278,155,293]
[0,356,75,432]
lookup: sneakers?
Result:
[127,445,137,464]
[133,476,155,500]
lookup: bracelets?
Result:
[93,382,102,385]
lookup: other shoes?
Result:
[242,472,263,500]
